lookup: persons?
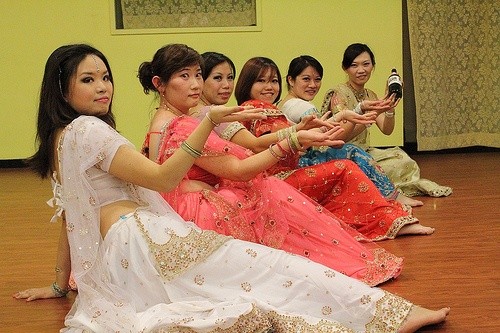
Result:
[186,51,339,154]
[321,43,453,198]
[13,44,451,333]
[138,44,405,288]
[235,57,435,241]
[276,55,424,208]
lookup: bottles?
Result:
[388,69,402,98]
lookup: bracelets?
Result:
[320,111,347,133]
[353,100,366,116]
[179,141,202,159]
[55,265,71,273]
[206,112,220,128]
[276,125,297,141]
[385,109,395,118]
[50,282,68,295]
[268,133,304,161]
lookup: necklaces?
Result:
[349,83,366,102]
